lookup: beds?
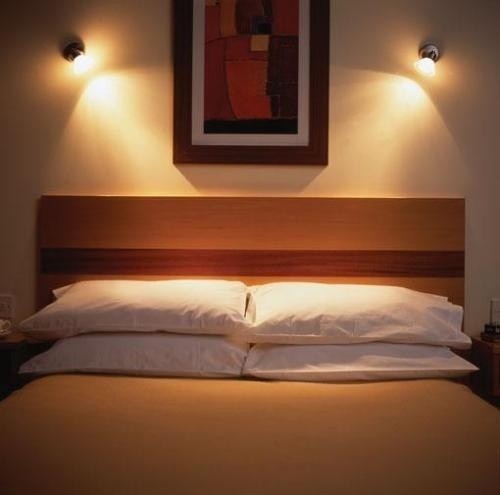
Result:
[3,194,499,495]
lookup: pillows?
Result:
[243,342,478,379]
[21,333,250,379]
[21,279,252,342]
[235,281,473,351]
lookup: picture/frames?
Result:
[168,2,332,166]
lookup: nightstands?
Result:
[468,327,499,399]
